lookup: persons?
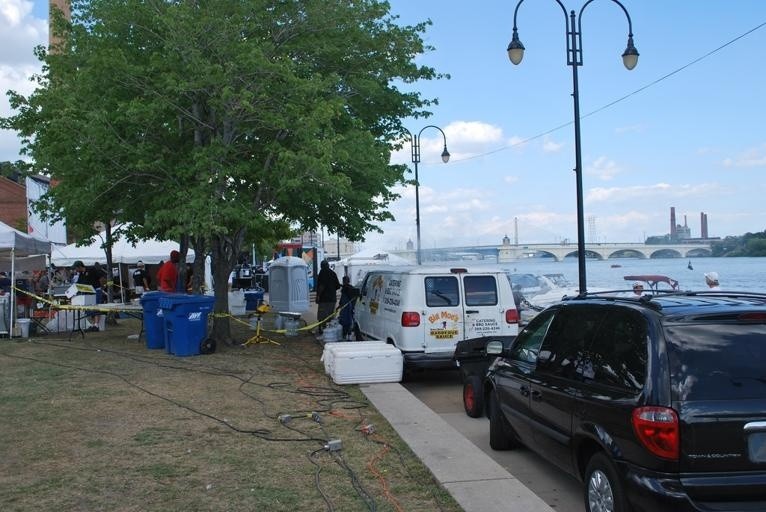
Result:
[627,281,647,298]
[39,270,48,292]
[72,261,104,332]
[186,262,194,292]
[315,260,344,334]
[51,263,59,279]
[155,250,179,292]
[133,261,150,319]
[145,267,151,285]
[704,272,720,288]
[336,275,360,341]
[94,261,108,278]
[0,271,10,292]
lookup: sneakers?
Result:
[84,326,99,331]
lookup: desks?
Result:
[28,315,55,335]
[60,305,144,344]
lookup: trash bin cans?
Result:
[244,291,264,320]
[15,318,31,337]
[139,291,217,357]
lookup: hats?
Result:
[171,251,179,257]
[137,262,143,266]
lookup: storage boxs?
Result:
[65,283,97,306]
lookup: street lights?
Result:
[507,0,640,300]
[410,125,451,264]
